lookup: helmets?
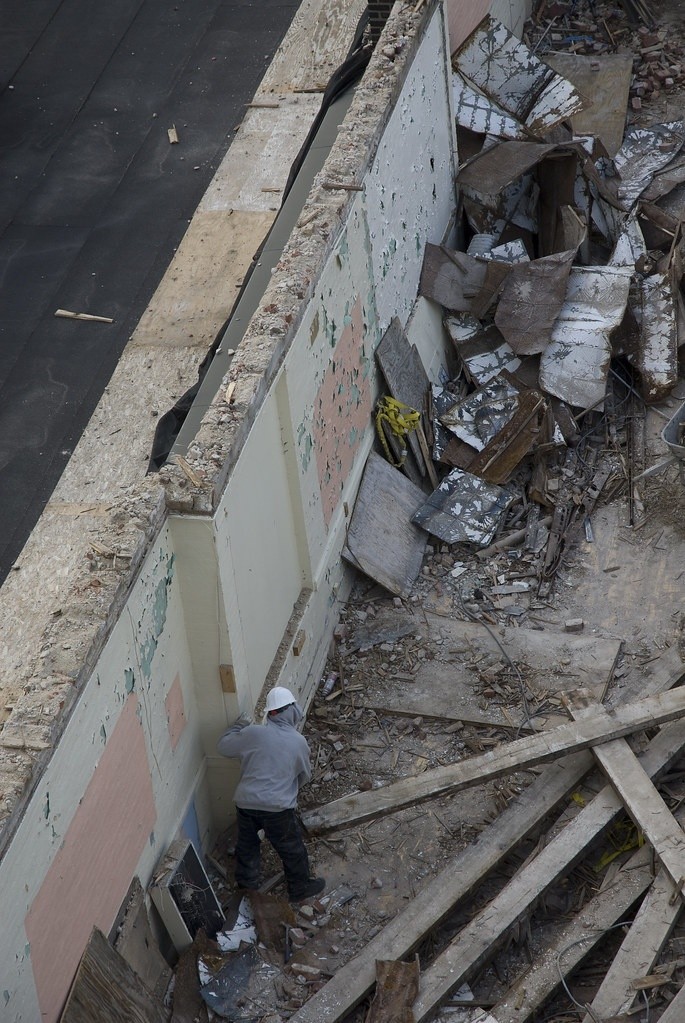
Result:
[263,687,297,711]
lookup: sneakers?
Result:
[238,877,266,892]
[288,877,325,903]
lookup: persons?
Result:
[215,686,326,903]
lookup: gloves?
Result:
[235,711,254,728]
